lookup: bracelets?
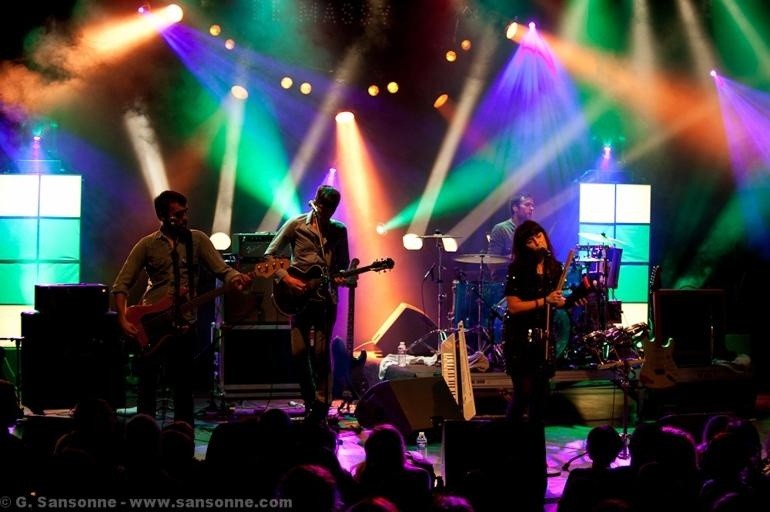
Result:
[544,296,546,308]
[536,300,538,310]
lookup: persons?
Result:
[558,415,770,512]
[487,192,536,278]
[503,220,567,415]
[110,190,252,421]
[265,186,349,408]
[0,379,435,512]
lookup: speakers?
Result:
[20,312,126,410]
[224,258,289,324]
[356,376,462,445]
[439,418,548,503]
[652,288,726,367]
[372,303,439,356]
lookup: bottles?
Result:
[396,341,410,367]
[413,431,428,460]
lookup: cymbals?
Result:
[449,253,513,264]
[576,232,629,246]
[415,234,462,239]
[575,258,603,264]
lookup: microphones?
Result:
[539,248,552,258]
[424,262,436,280]
[309,199,318,213]
[435,229,449,256]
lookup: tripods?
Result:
[455,264,507,373]
[404,248,475,354]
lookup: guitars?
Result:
[123,252,291,355]
[269,258,395,318]
[637,263,679,390]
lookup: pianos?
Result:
[440,351,458,405]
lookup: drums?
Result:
[490,293,569,367]
[453,279,507,332]
[590,245,623,289]
[560,261,582,289]
